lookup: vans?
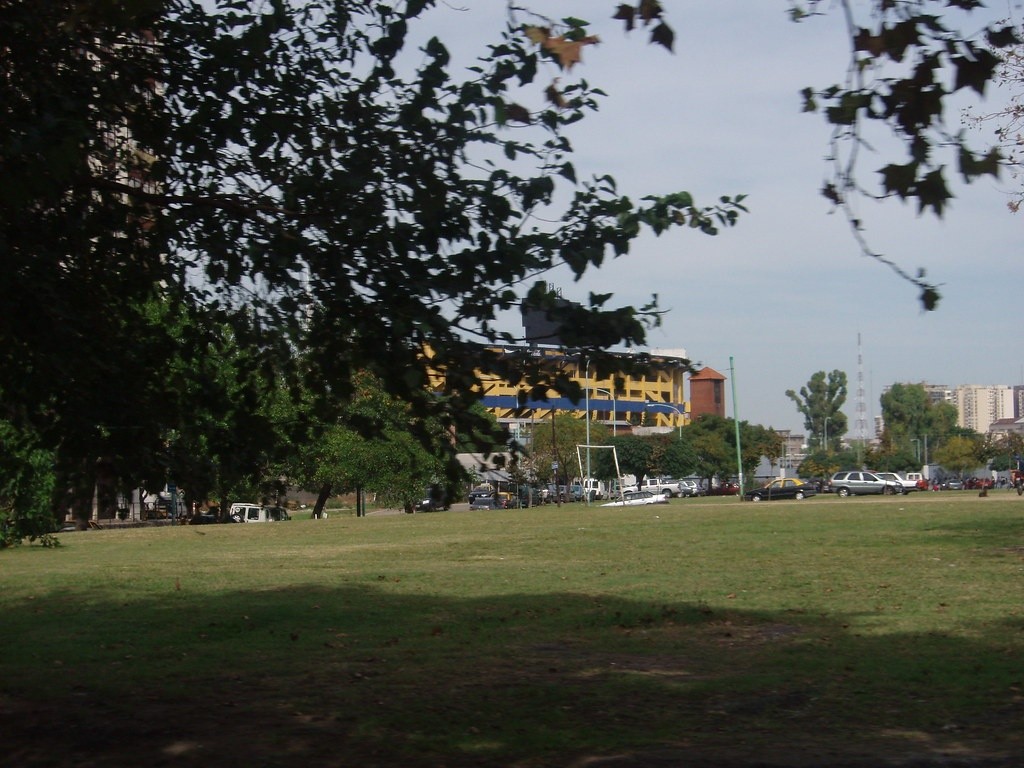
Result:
[231,503,292,524]
[905,472,924,493]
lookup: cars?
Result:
[468,485,497,504]
[743,477,818,503]
[58,519,103,532]
[486,479,749,510]
[947,478,964,490]
[874,471,919,496]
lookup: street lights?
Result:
[911,438,921,463]
[581,388,616,437]
[648,404,682,439]
[824,416,831,452]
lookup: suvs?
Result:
[405,486,449,515]
[825,470,903,499]
